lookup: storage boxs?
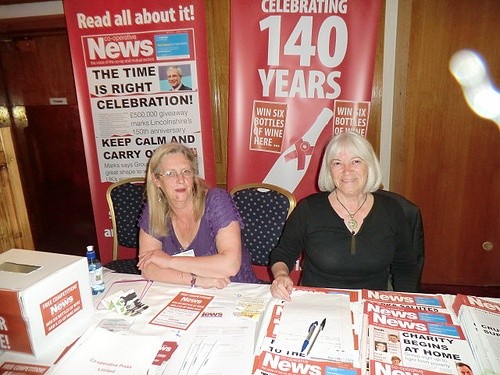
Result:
[0,248,92,365]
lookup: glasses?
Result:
[157,168,194,178]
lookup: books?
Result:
[252,289,500,375]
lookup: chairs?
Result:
[227,184,296,285]
[104,178,147,275]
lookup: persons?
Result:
[391,356,401,365]
[456,361,474,375]
[166,66,192,91]
[375,341,387,352]
[269,130,419,301]
[388,334,400,344]
[137,142,256,289]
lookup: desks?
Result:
[0,272,500,375]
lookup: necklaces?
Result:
[334,188,367,234]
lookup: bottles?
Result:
[87,246,105,295]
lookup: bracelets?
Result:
[191,273,196,288]
